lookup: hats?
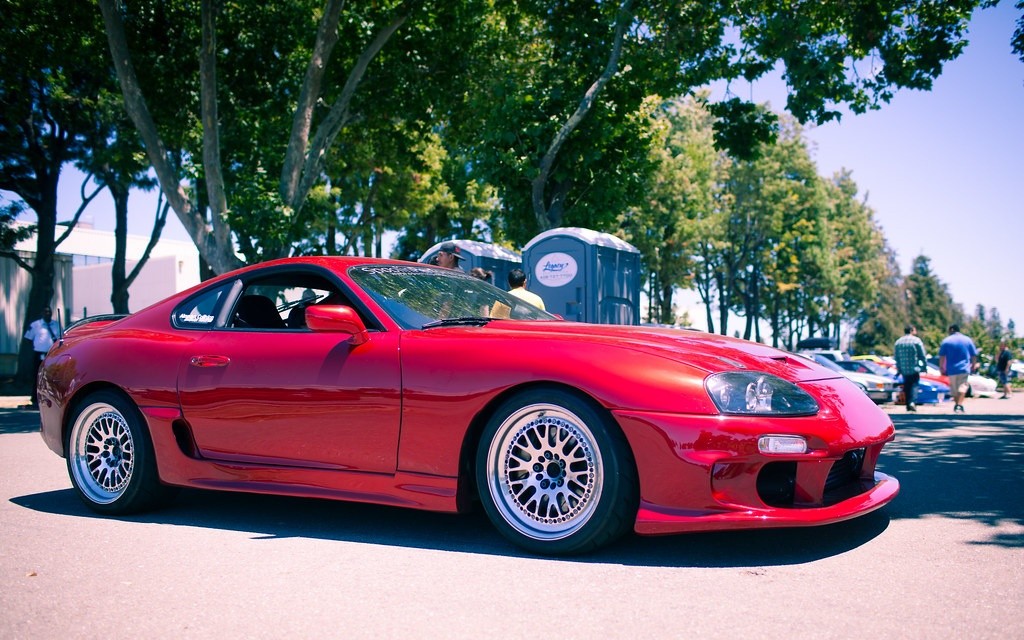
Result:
[439,243,466,261]
[472,267,487,282]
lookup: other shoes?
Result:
[954,405,964,414]
[1000,396,1008,399]
[909,402,916,411]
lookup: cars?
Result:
[790,336,999,405]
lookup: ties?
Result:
[47,324,57,344]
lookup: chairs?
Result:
[236,295,289,328]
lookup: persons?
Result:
[939,324,977,413]
[996,344,1013,399]
[299,289,316,304]
[490,269,546,319]
[436,244,466,321]
[894,324,927,411]
[470,267,494,316]
[22,307,62,410]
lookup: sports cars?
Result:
[36,255,899,560]
